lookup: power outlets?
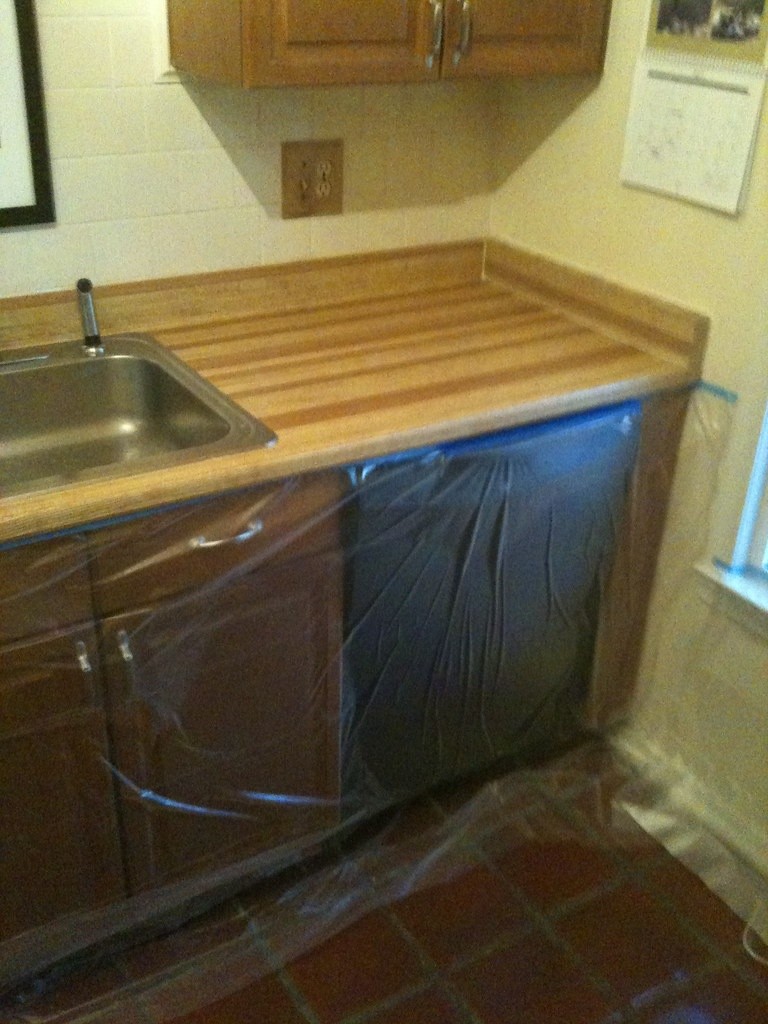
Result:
[280,138,344,220]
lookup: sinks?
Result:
[0,342,279,506]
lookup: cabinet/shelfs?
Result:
[0,464,346,996]
[168,0,614,92]
